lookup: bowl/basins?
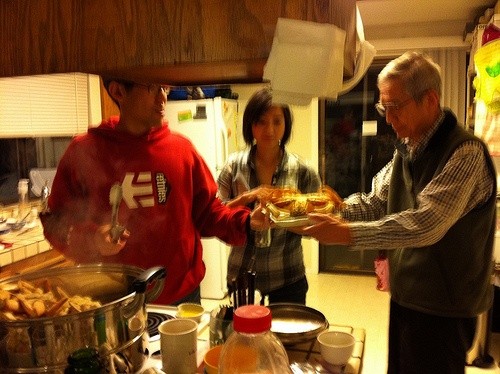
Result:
[257,197,342,228]
[204,345,222,374]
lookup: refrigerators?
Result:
[159,97,248,300]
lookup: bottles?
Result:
[218,304,291,374]
[17,178,29,214]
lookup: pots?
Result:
[0,261,168,374]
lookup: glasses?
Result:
[134,85,170,96]
[375,88,432,118]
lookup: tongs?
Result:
[108,183,126,244]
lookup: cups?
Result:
[209,305,233,349]
[316,330,356,365]
[157,318,198,374]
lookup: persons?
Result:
[216,86,322,308]
[43,71,272,304]
[288,50,496,373]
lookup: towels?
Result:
[261,17,347,109]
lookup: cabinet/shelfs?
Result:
[0,0,358,86]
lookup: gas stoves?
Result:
[146,301,209,370]
